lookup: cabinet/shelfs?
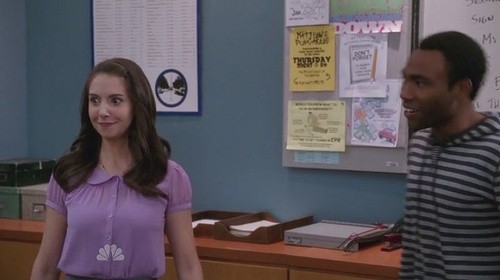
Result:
[1,211,402,278]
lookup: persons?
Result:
[29,56,204,280]
[397,30,500,279]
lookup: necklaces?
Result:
[99,158,110,177]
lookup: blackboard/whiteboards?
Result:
[410,0,500,122]
